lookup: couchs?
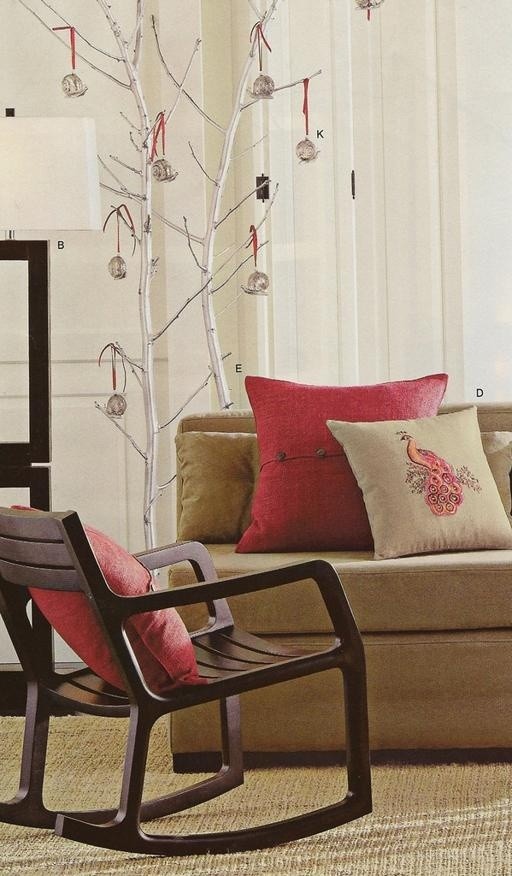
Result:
[169,403,511,773]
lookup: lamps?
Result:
[1,117,102,241]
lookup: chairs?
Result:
[2,507,375,857]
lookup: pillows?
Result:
[325,406,509,559]
[236,375,451,554]
[13,505,208,697]
